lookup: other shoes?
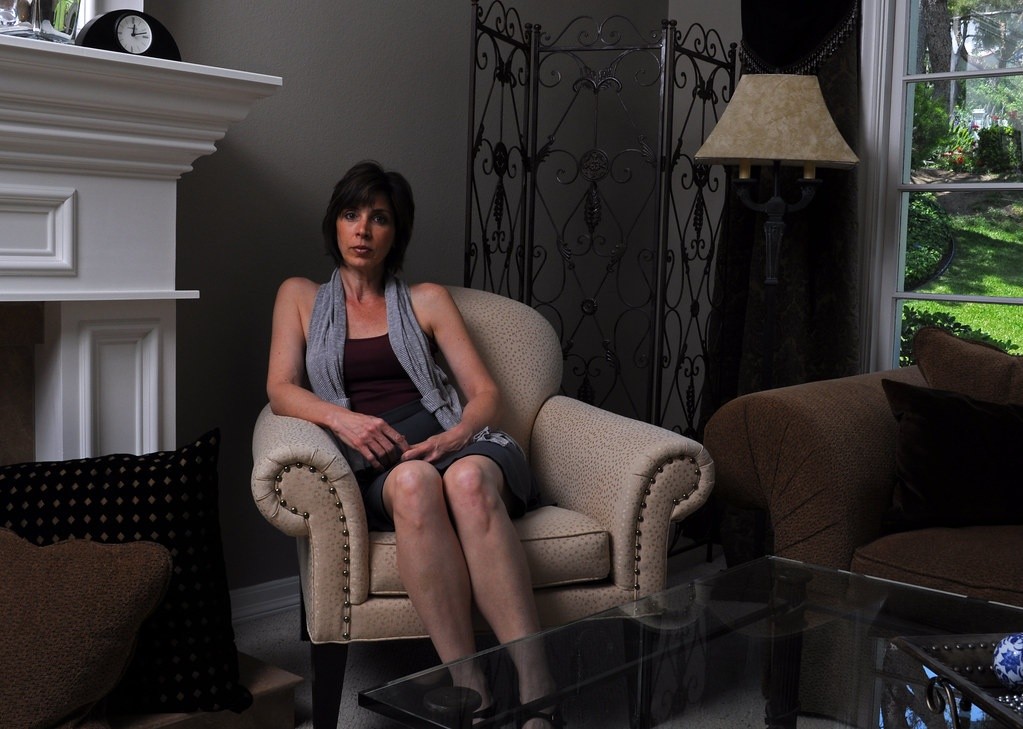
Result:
[472,698,497,729]
[518,698,567,728]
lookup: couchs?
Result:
[705,363,1023,729]
[252,284,717,729]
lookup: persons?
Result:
[267,158,560,728]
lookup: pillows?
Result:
[0,428,259,717]
[0,528,170,729]
[914,328,1022,403]
[879,376,1023,535]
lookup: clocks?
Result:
[115,13,152,54]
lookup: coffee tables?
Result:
[356,555,1023,729]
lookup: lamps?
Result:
[693,75,861,387]
